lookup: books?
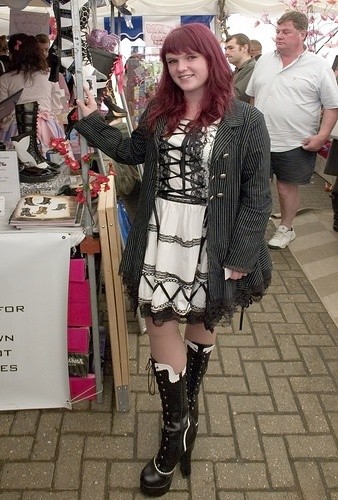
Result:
[8,194,86,230]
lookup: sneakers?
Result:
[268,225,296,249]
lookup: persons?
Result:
[0,29,145,152]
[226,10,338,249]
[73,25,276,496]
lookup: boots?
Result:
[141,353,196,497]
[11,101,60,182]
[329,192,338,232]
[86,46,127,116]
[186,339,214,426]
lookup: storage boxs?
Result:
[67,258,99,399]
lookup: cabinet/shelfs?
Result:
[0,0,122,413]
